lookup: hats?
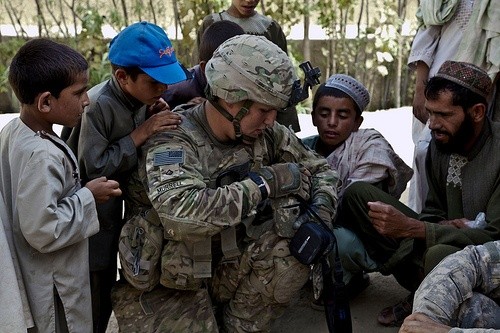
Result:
[106,21,188,84]
[435,60,493,96]
[324,74,370,113]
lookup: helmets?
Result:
[205,34,299,111]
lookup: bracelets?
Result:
[246,172,267,204]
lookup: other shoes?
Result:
[312,289,365,311]
[377,294,415,326]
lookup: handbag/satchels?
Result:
[159,239,203,291]
[118,209,164,316]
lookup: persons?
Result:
[163,21,244,111]
[295,74,414,311]
[397,239,500,333]
[0,39,121,332]
[338,60,500,326]
[197,0,302,138]
[406,0,499,218]
[111,32,342,333]
[61,20,188,333]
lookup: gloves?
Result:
[293,208,334,232]
[261,161,311,201]
